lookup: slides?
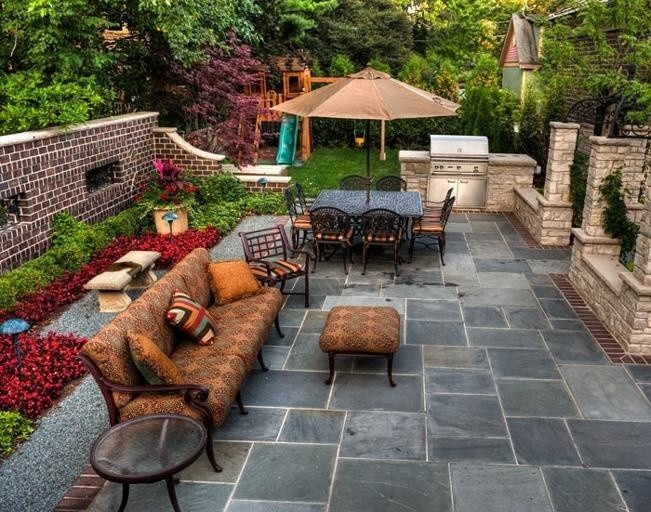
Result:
[276,112,299,165]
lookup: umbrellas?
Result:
[267,66,462,207]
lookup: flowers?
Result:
[136,158,194,203]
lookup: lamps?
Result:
[353,129,366,148]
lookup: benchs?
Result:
[83,250,161,314]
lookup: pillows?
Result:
[204,259,264,305]
[125,328,182,392]
[164,288,216,347]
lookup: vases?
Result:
[150,205,187,235]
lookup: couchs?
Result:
[81,247,284,472]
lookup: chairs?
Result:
[238,224,315,308]
[283,176,456,276]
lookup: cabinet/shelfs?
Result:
[428,176,486,210]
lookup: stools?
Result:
[318,305,399,388]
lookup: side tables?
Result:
[91,415,210,512]
